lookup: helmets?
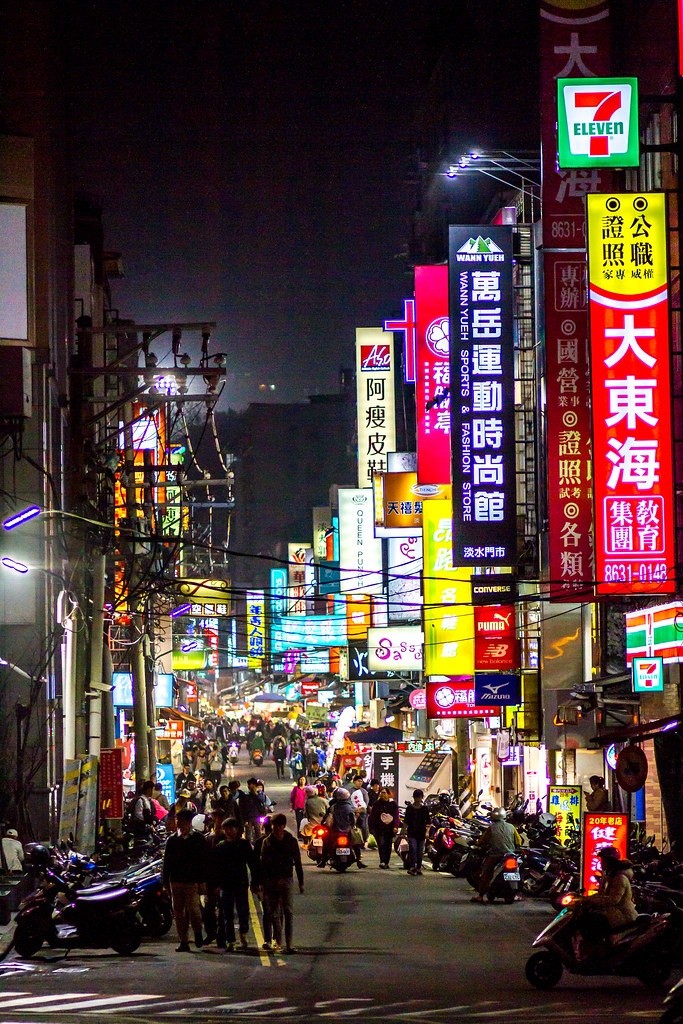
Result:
[192,814,207,832]
[333,787,350,800]
[491,806,507,820]
[306,783,326,798]
[30,844,50,864]
[255,731,262,737]
[597,847,620,862]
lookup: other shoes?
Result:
[576,957,603,969]
[379,862,390,869]
[407,868,422,876]
[357,860,367,869]
[470,897,484,904]
[174,926,297,954]
[317,861,326,868]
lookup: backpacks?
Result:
[125,797,146,818]
[210,759,222,771]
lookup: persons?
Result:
[288,761,381,869]
[183,714,330,791]
[132,762,306,952]
[577,846,638,942]
[470,807,522,904]
[432,790,460,871]
[583,775,608,812]
[369,786,400,869]
[405,789,432,876]
[2,830,24,874]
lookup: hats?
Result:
[179,788,191,799]
[7,829,18,837]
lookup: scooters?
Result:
[13,837,174,960]
[252,801,278,842]
[237,721,249,743]
[390,786,682,914]
[319,811,358,873]
[659,976,683,1024]
[250,741,264,767]
[524,885,683,989]
[226,741,239,766]
[299,809,329,865]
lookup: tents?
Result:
[349,726,415,776]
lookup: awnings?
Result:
[250,694,290,712]
[574,672,642,703]
[556,692,598,724]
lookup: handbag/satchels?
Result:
[350,825,364,848]
[397,839,409,853]
[296,760,302,770]
[351,789,367,809]
